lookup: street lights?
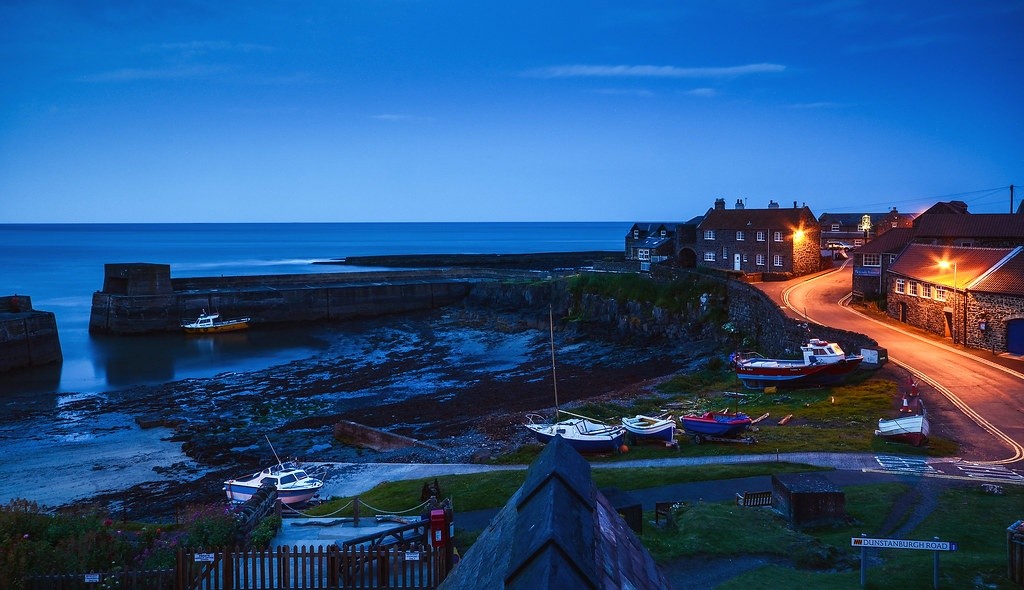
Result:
[863,221,871,244]
[796,231,805,277]
[939,260,956,343]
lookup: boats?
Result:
[621,414,677,443]
[180,294,252,333]
[732,307,864,390]
[223,435,323,509]
[878,376,930,446]
[524,410,626,450]
[681,411,753,437]
